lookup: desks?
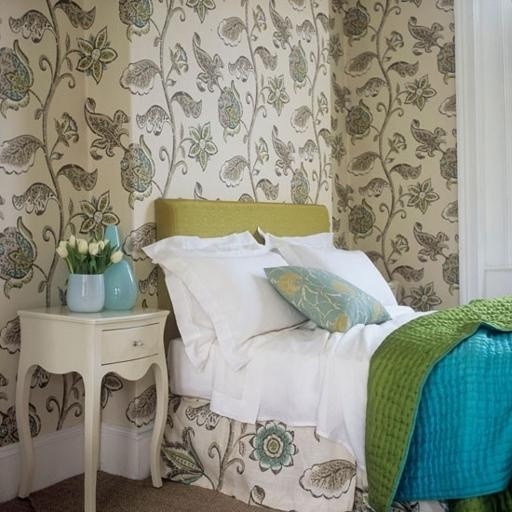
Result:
[14,307,171,511]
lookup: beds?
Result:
[156,198,511,510]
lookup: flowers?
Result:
[56,235,123,274]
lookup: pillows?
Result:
[142,229,262,371]
[275,241,398,308]
[264,266,392,333]
[257,226,334,250]
[152,249,310,373]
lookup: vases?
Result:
[105,224,137,311]
[66,274,105,312]
